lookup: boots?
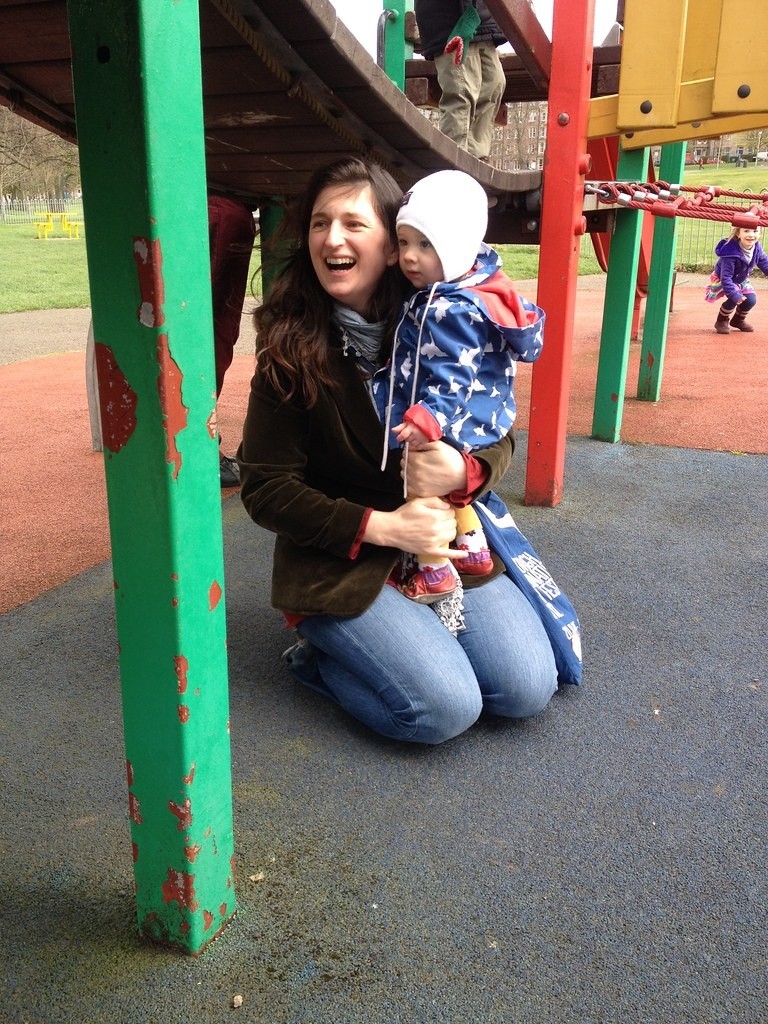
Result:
[730,307,754,332]
[714,302,735,334]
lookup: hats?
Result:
[395,170,488,282]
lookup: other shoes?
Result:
[281,643,299,664]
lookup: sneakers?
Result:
[219,452,240,487]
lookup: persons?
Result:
[413,0,512,163]
[235,150,560,746]
[383,168,547,605]
[703,225,768,334]
[209,192,254,489]
[699,158,704,169]
